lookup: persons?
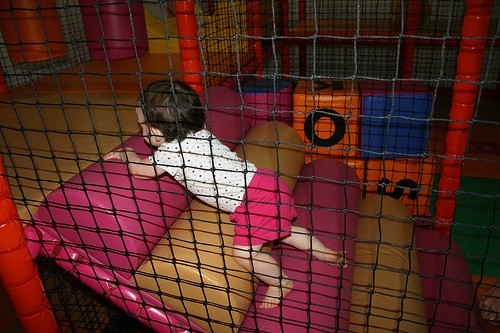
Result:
[102,79,348,309]
